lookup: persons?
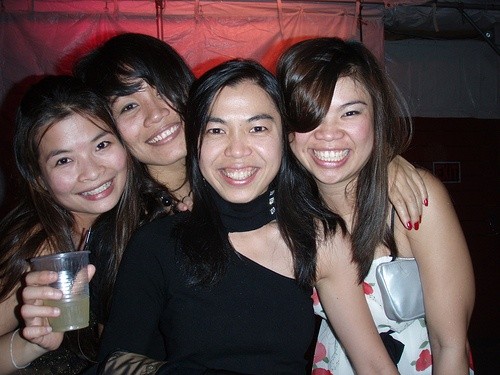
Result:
[0,74,135,375]
[77,31,198,217]
[276,37,475,375]
[99,57,399,375]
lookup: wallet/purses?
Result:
[376,260,425,321]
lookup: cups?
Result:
[30,250,90,333]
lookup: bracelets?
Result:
[10,328,31,369]
